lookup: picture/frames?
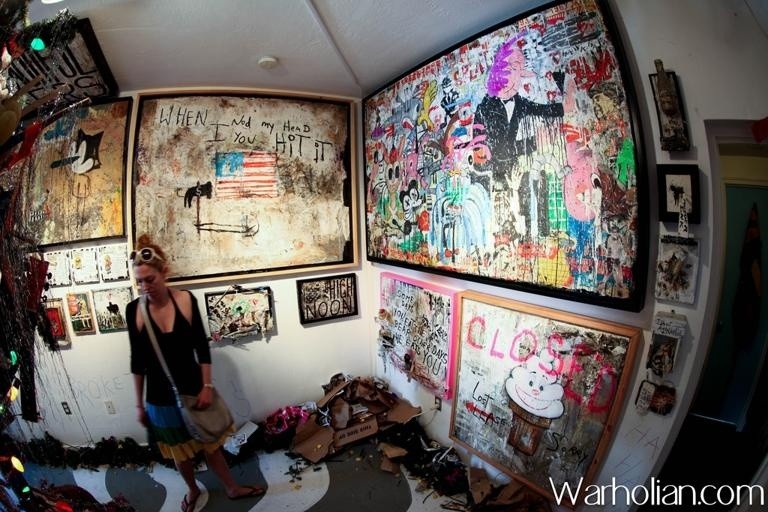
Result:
[354,0,649,316]
[7,17,121,107]
[125,87,361,294]
[296,271,359,325]
[654,164,700,223]
[204,286,276,340]
[447,286,644,509]
[8,95,132,249]
[376,271,460,401]
[648,60,693,152]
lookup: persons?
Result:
[124,232,266,512]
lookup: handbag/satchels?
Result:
[176,388,233,444]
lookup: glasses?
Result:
[130,248,162,265]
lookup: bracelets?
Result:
[203,383,214,388]
[136,405,145,409]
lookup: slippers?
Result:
[181,490,200,512]
[228,485,266,500]
